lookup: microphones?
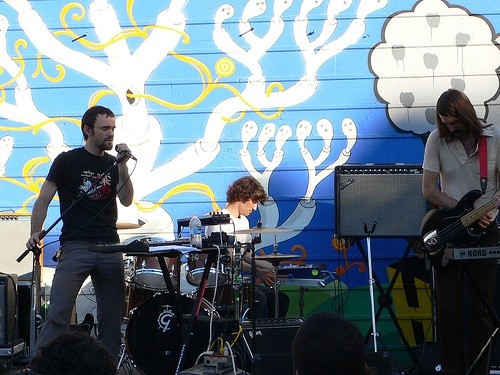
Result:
[115,144,136,161]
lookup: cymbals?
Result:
[253,253,302,261]
[228,228,294,235]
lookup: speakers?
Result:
[239,318,304,375]
[335,166,434,237]
[18,281,31,346]
[0,273,18,347]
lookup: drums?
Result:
[125,293,223,375]
[185,253,226,287]
[131,255,180,291]
[191,286,237,318]
[123,271,166,321]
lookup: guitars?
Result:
[420,190,500,269]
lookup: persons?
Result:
[292,312,375,375]
[421,89,500,375]
[26,106,134,359]
[202,176,277,319]
[28,330,117,375]
